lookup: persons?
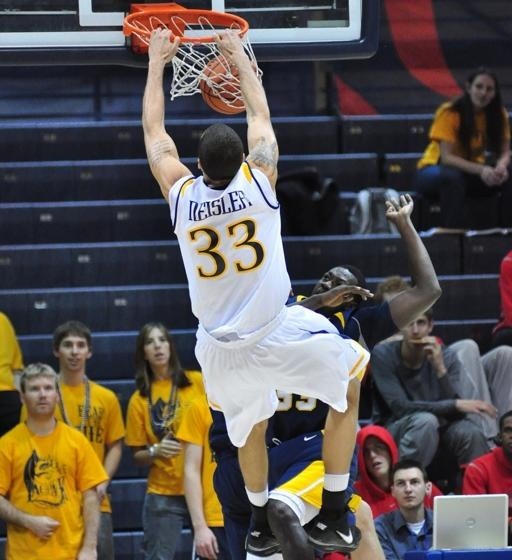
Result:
[0,317,229,560]
[208,263,374,560]
[206,193,442,560]
[355,252,512,560]
[413,68,511,235]
[143,27,373,560]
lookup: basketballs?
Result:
[200,53,264,116]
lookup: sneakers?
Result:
[308,518,362,552]
[247,528,283,556]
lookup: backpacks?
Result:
[349,186,400,235]
